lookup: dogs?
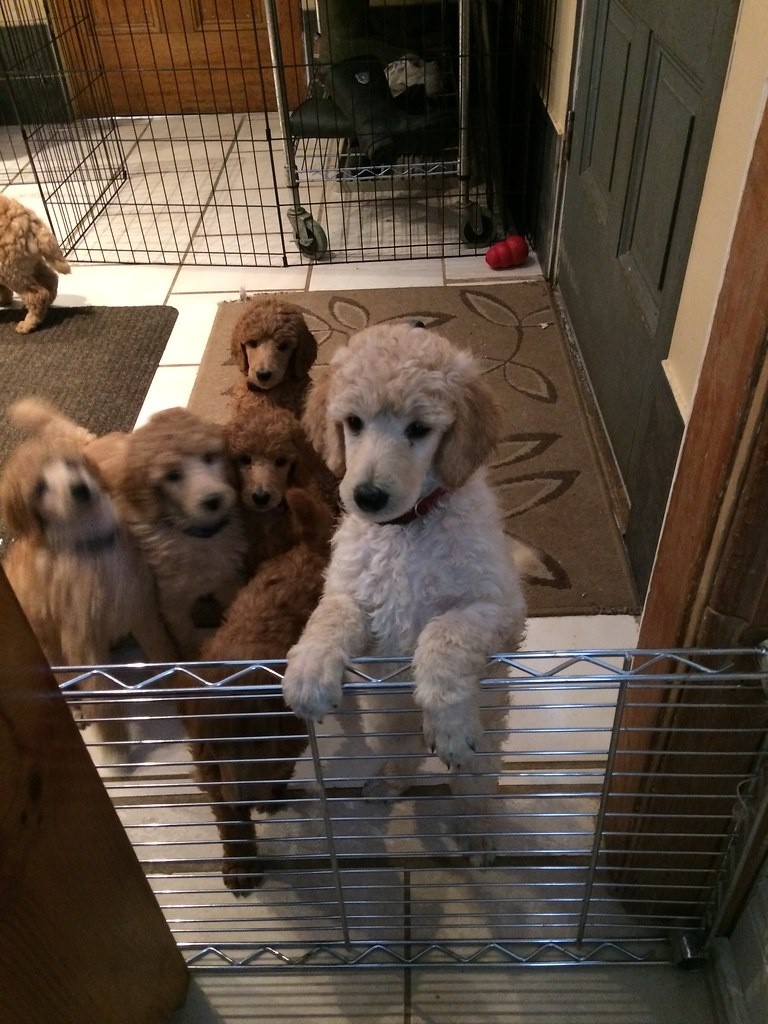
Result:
[280,324,527,862]
[174,494,328,895]
[231,296,316,427]
[225,406,322,545]
[0,398,173,780]
[0,195,71,335]
[120,405,248,651]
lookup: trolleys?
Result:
[265,0,500,259]
